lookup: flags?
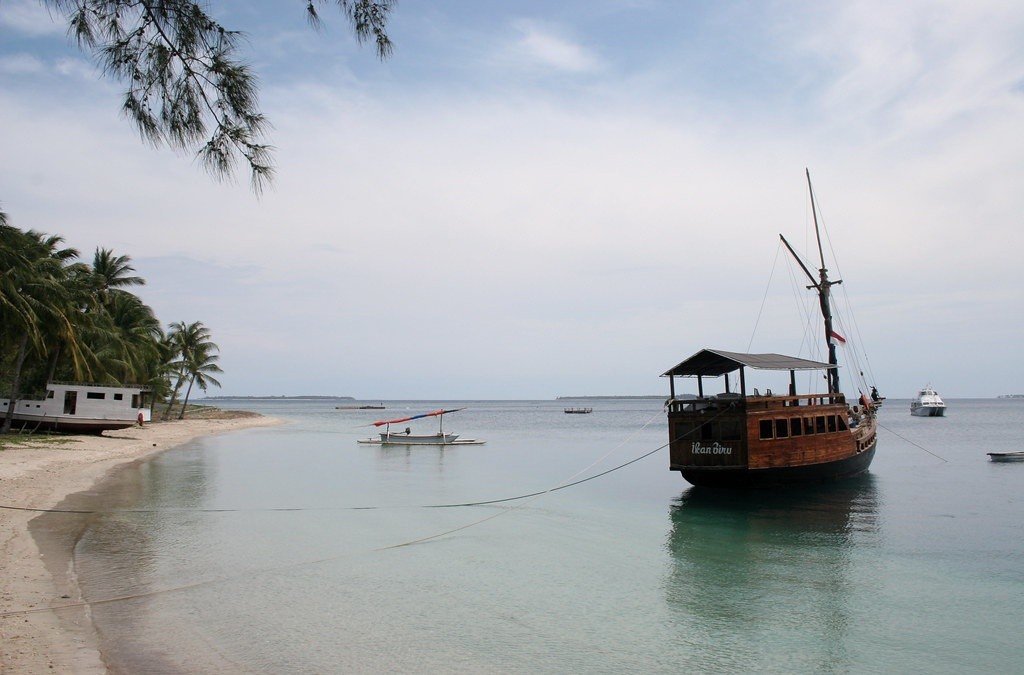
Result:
[828,330,847,349]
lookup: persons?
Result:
[846,403,867,428]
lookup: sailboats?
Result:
[659,165,887,484]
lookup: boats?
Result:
[985,449,1023,463]
[563,407,594,414]
[358,406,486,445]
[0,384,153,432]
[910,381,947,416]
